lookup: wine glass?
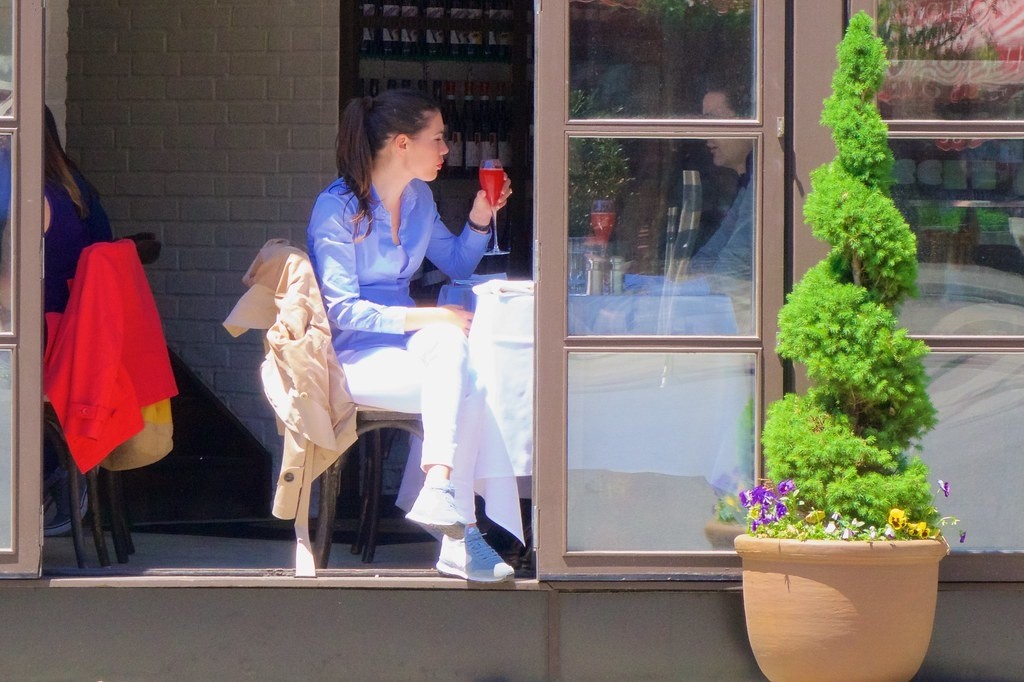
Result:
[479,159,510,256]
[591,200,616,295]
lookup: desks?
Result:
[396,274,751,564]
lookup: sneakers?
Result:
[436,526,514,584]
[407,479,466,540]
[42,420,99,536]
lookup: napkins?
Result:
[472,278,535,300]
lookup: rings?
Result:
[509,188,512,193]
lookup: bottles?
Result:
[588,261,604,295]
[610,255,625,295]
[360,77,516,178]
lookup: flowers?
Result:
[739,482,968,547]
[713,474,744,527]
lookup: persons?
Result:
[305,91,514,582]
[685,72,753,303]
[0,88,111,537]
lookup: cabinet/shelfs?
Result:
[339,1,615,182]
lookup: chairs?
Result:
[253,238,534,570]
[45,232,162,569]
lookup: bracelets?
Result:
[468,216,491,230]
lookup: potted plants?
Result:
[732,7,945,682]
[704,384,759,551]
